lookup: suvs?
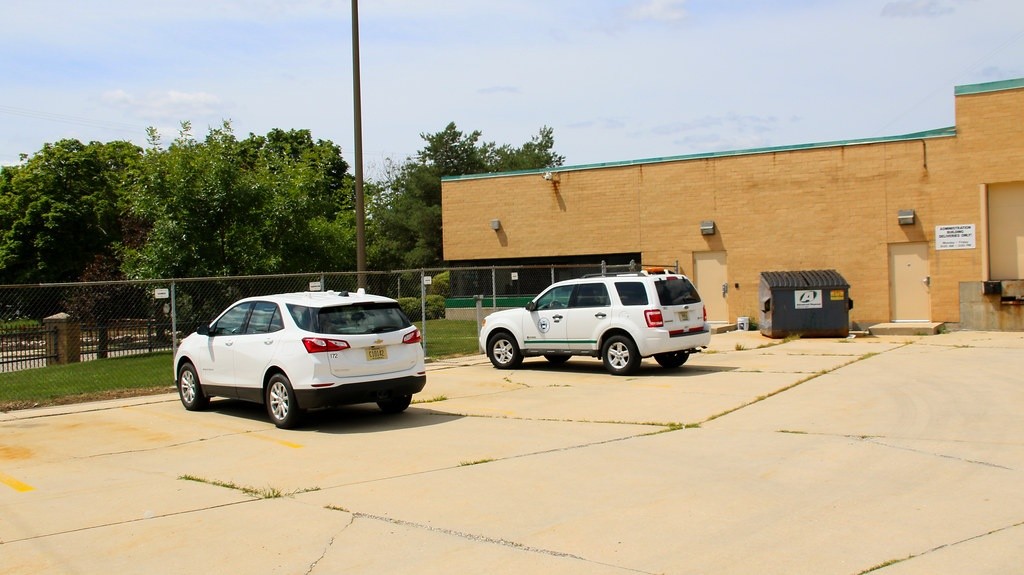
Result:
[173,287,428,430]
[477,269,712,377]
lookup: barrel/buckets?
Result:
[737,316,749,331]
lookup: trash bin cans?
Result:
[758,270,853,338]
